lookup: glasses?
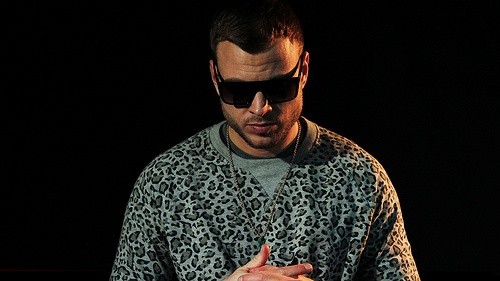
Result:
[213,50,306,108]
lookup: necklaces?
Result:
[226,119,302,243]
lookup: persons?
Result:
[108,0,422,281]
